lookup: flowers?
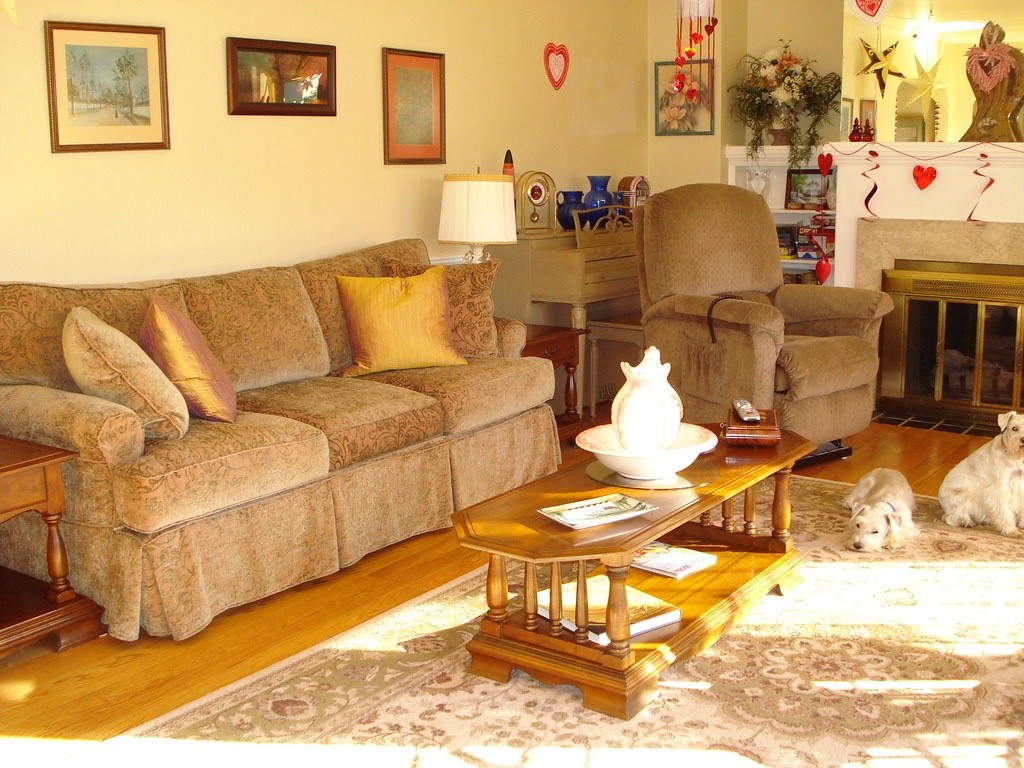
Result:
[727,38,842,170]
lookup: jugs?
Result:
[556,191,588,230]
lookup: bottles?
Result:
[862,118,875,142]
[611,192,625,225]
[849,118,862,142]
[584,176,613,227]
[746,169,772,198]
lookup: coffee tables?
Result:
[521,323,591,426]
[0,434,106,661]
[450,423,818,721]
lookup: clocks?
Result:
[516,171,557,230]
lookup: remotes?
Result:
[733,397,761,422]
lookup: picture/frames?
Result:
[654,58,714,136]
[842,97,854,142]
[785,169,833,210]
[860,100,877,141]
[226,37,336,117]
[44,20,171,153]
[382,47,446,165]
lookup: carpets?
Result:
[102,473,1024,768]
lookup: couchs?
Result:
[632,183,895,470]
[0,238,562,641]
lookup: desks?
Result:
[483,224,639,419]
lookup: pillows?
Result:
[62,306,189,440]
[140,296,237,424]
[334,264,467,379]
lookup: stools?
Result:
[586,312,646,417]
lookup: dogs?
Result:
[938,410,1024,537]
[841,467,916,553]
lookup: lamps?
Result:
[437,166,517,264]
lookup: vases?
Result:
[557,176,625,229]
[767,107,796,146]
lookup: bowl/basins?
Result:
[576,422,718,480]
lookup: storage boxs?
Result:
[775,220,835,260]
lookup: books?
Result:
[775,220,834,259]
[629,541,717,580]
[523,573,683,646]
